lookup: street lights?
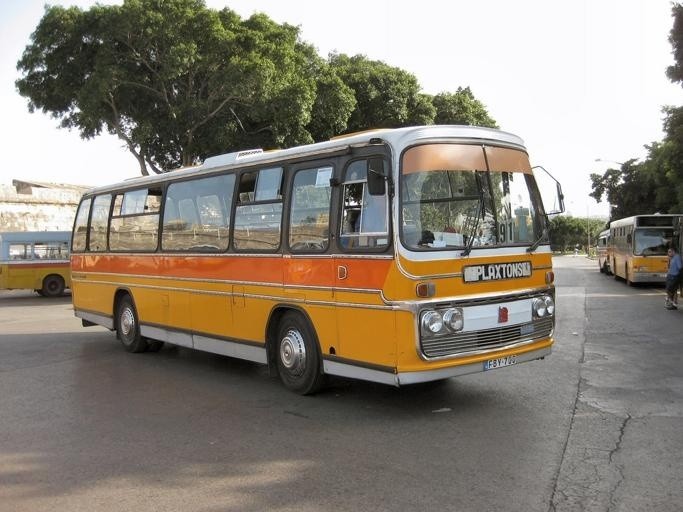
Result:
[593,157,624,166]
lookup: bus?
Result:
[608,211,682,287]
[320,223,415,252]
[0,229,71,298]
[61,123,572,402]
[594,228,613,276]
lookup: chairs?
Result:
[75,226,136,251]
[345,209,362,234]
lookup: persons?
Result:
[663,246,682,309]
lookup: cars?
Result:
[290,241,323,251]
